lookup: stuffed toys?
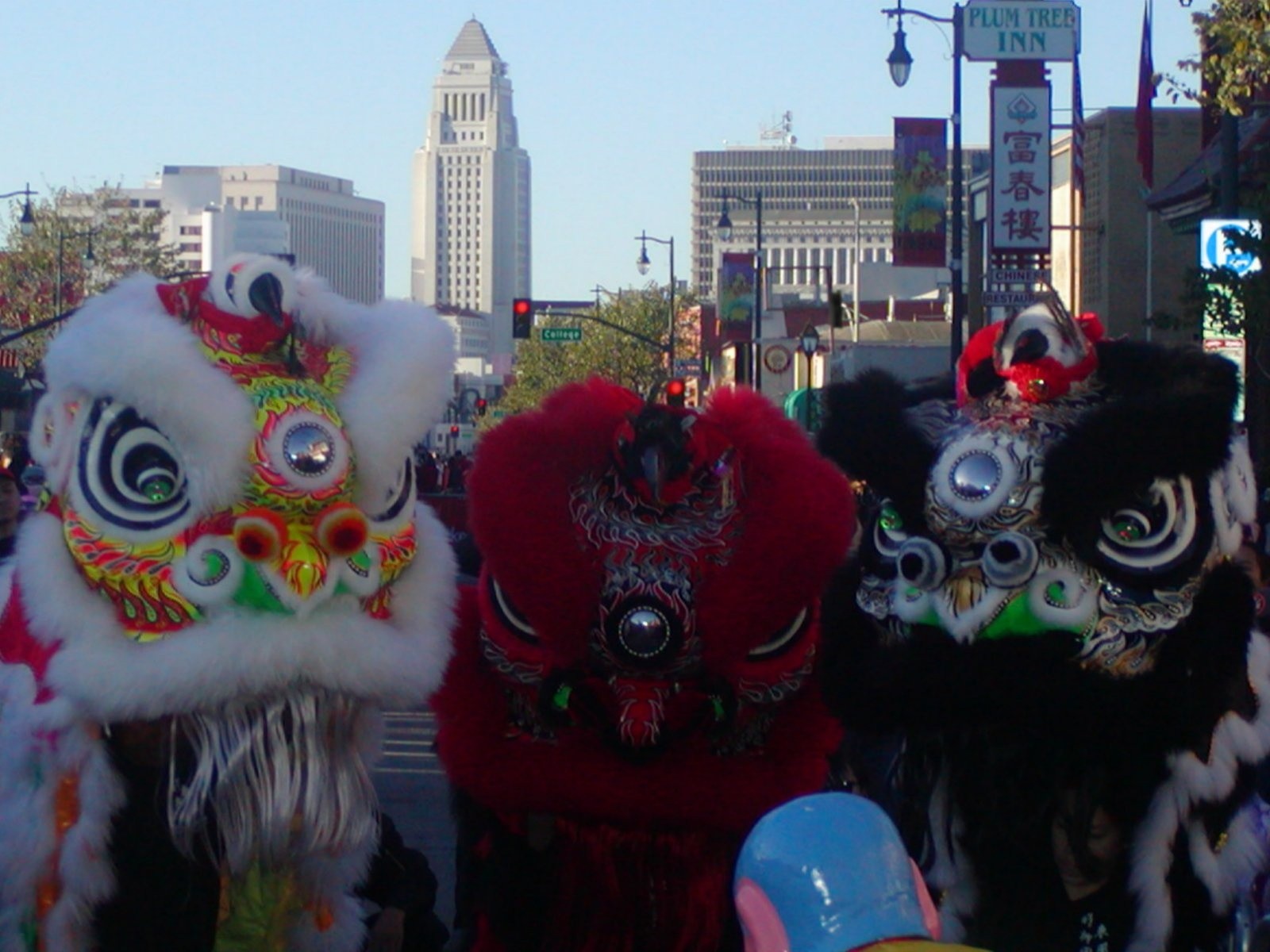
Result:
[817,306,1269,951]
[426,378,855,952]
[0,252,462,952]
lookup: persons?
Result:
[368,812,451,952]
[0,468,21,559]
[416,446,469,494]
[733,793,985,952]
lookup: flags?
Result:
[1074,56,1086,193]
[1202,51,1225,147]
[1136,17,1158,191]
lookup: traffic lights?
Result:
[666,377,686,410]
[512,298,533,339]
[450,425,459,437]
[476,398,487,418]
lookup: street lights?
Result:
[880,1,964,410]
[796,319,820,435]
[849,197,861,345]
[593,282,623,387]
[57,230,94,332]
[635,229,676,377]
[712,183,764,391]
